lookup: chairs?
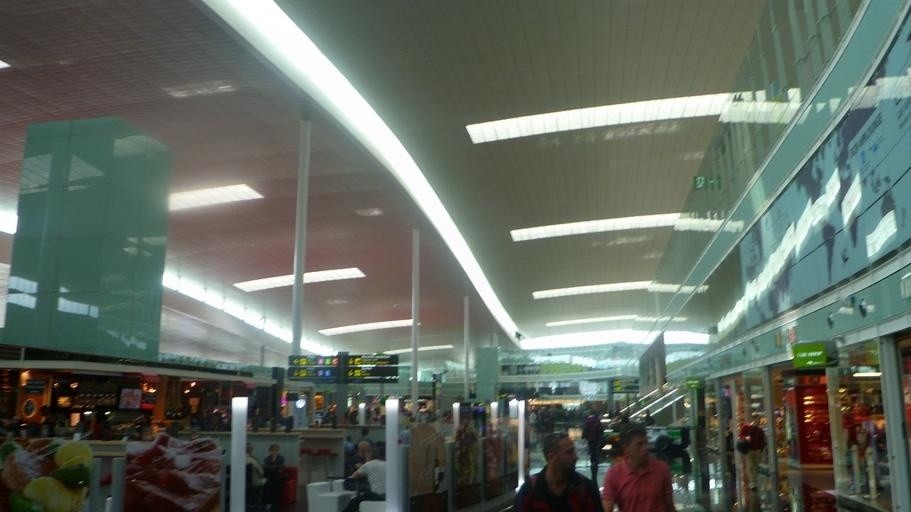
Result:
[263,466,389,512]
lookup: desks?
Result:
[299,448,338,483]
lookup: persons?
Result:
[247,442,286,510]
[344,426,409,511]
[1,407,114,441]
[740,415,764,490]
[515,405,676,511]
[251,407,261,428]
[323,407,381,425]
[131,407,231,441]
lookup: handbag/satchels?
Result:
[737,438,750,453]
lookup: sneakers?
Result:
[749,482,756,489]
